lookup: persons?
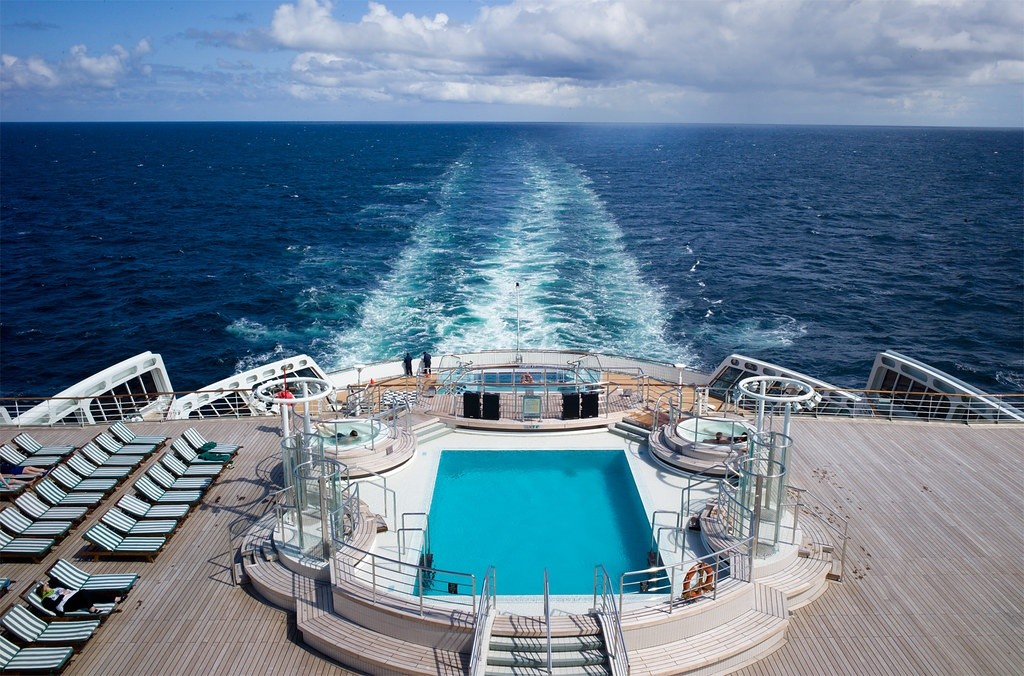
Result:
[423,352,431,374]
[703,432,732,444]
[734,432,747,443]
[36,584,126,614]
[0,461,45,476]
[314,422,357,437]
[0,473,35,491]
[404,353,412,376]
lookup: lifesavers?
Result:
[683,563,713,599]
[521,374,533,384]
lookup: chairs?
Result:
[0,421,243,676]
